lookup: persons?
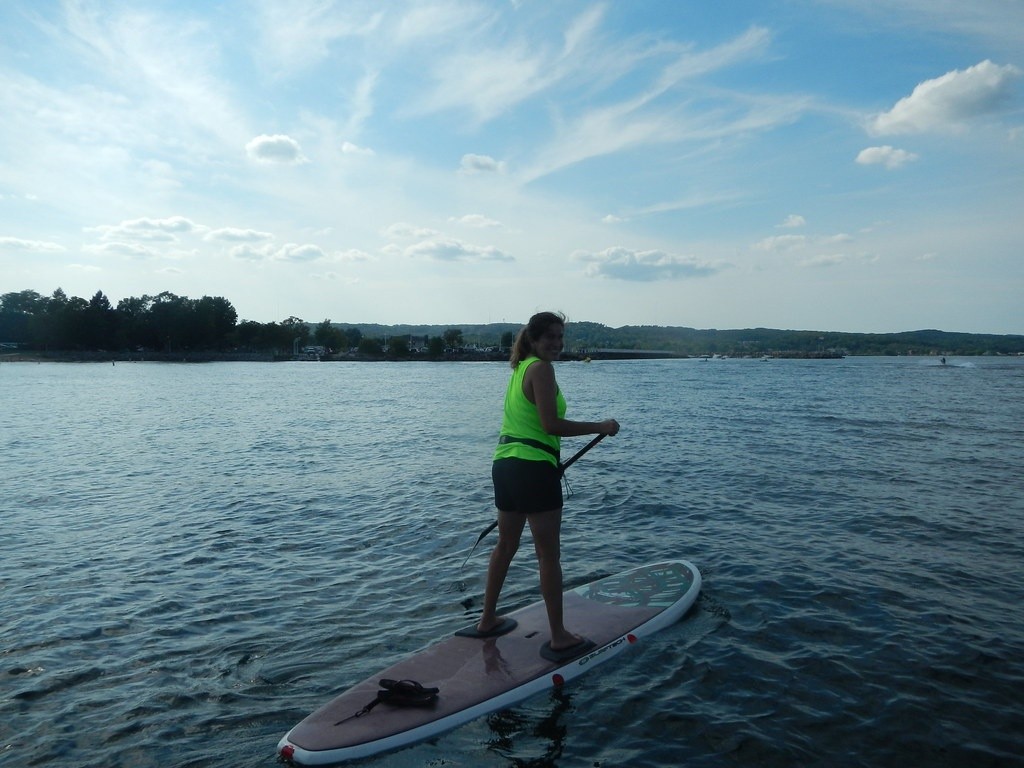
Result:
[479,312,621,650]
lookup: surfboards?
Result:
[277,559,701,767]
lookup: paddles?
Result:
[455,419,608,571]
